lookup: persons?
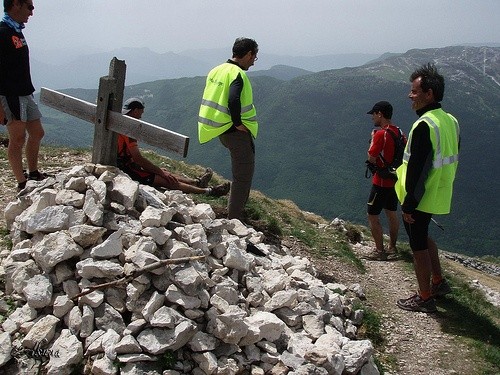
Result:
[0,0,56,193]
[118,99,231,196]
[395,64,460,312]
[198,38,259,226]
[363,102,407,262]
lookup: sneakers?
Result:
[382,244,398,254]
[361,249,387,261]
[429,278,453,298]
[396,290,438,313]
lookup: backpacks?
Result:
[364,127,405,180]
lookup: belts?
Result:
[220,128,236,136]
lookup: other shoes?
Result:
[17,185,27,193]
[28,172,47,181]
[197,166,213,188]
[210,181,231,197]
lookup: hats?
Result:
[366,100,394,115]
[121,97,145,115]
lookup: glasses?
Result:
[254,54,259,61]
[23,1,35,11]
